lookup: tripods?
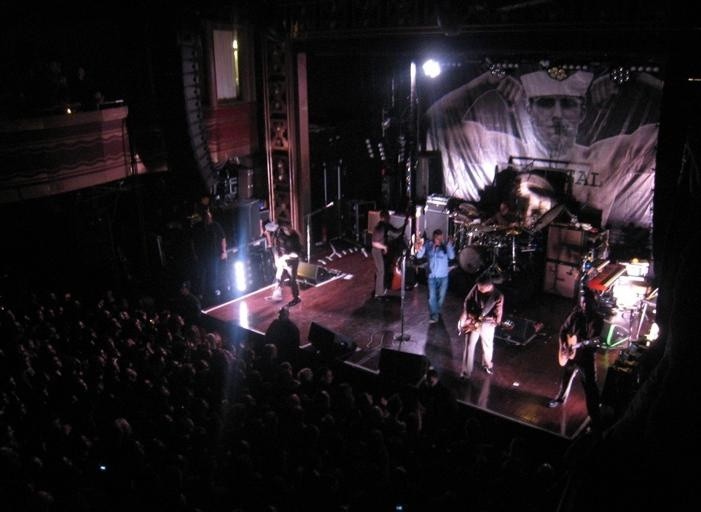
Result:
[475,238,512,285]
[329,159,363,247]
[314,162,342,258]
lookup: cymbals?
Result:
[478,224,509,232]
[459,202,478,215]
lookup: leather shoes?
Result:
[483,367,493,375]
[375,295,392,302]
[264,297,280,304]
[428,314,445,324]
[288,297,302,306]
[460,372,470,379]
[547,400,561,408]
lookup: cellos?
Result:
[374,204,414,292]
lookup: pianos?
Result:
[586,264,627,291]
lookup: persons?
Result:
[370,210,406,298]
[264,223,301,306]
[190,207,227,296]
[1,278,551,511]
[415,229,456,323]
[460,273,505,379]
[425,68,663,228]
[482,202,521,227]
[547,294,604,416]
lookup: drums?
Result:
[457,239,494,275]
[454,214,471,226]
[496,242,521,262]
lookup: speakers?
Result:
[296,262,327,284]
[378,348,430,385]
[308,322,356,354]
[541,223,590,301]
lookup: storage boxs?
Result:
[543,262,579,300]
[367,209,413,236]
[546,223,589,266]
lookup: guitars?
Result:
[558,333,603,367]
[457,312,515,334]
